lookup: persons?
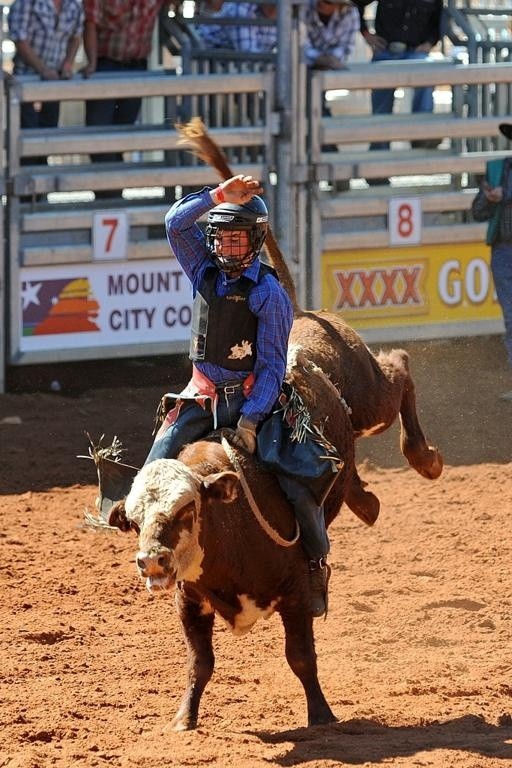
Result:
[79,0,163,201]
[358,1,445,188]
[472,122,512,405]
[182,0,298,157]
[106,174,333,618]
[6,0,86,203]
[298,2,360,192]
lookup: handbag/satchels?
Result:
[473,193,496,223]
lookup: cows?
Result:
[106,115,444,732]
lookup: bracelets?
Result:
[214,186,225,202]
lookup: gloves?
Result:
[231,416,260,453]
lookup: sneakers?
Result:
[308,567,328,616]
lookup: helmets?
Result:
[204,194,270,272]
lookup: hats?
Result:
[499,120,511,139]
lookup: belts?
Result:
[215,383,241,396]
[390,42,414,53]
